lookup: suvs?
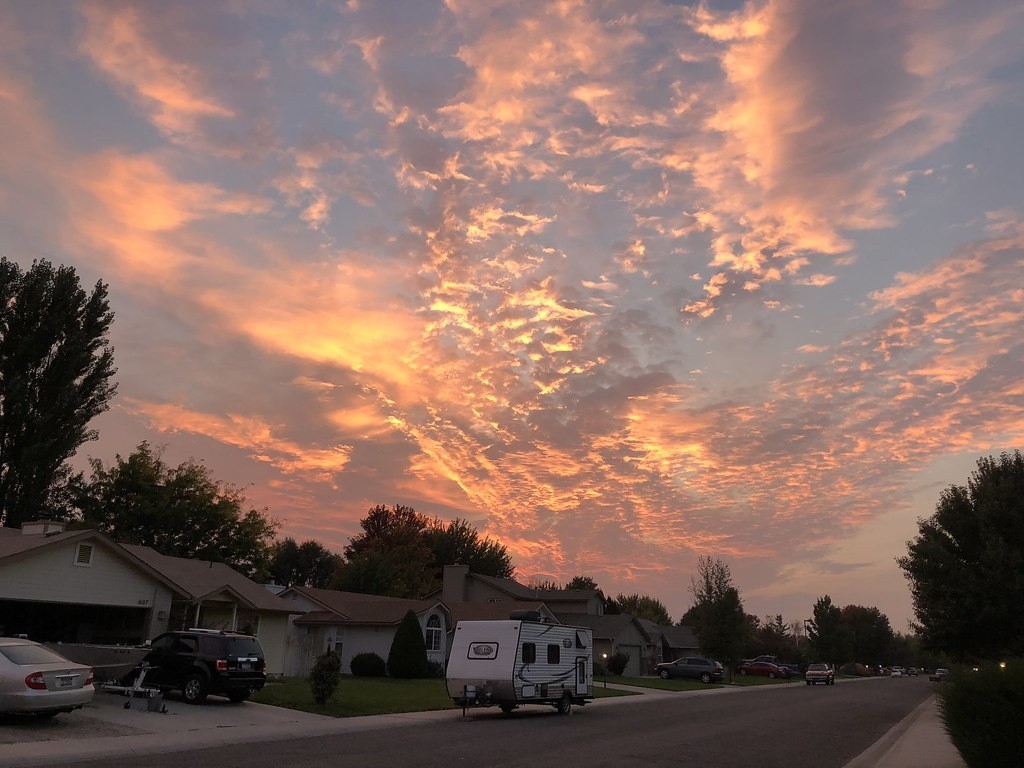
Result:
[656,656,726,685]
[115,628,267,705]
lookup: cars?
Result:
[0,636,95,723]
[806,663,835,686]
[736,654,805,680]
[930,669,950,681]
[867,664,924,678]
